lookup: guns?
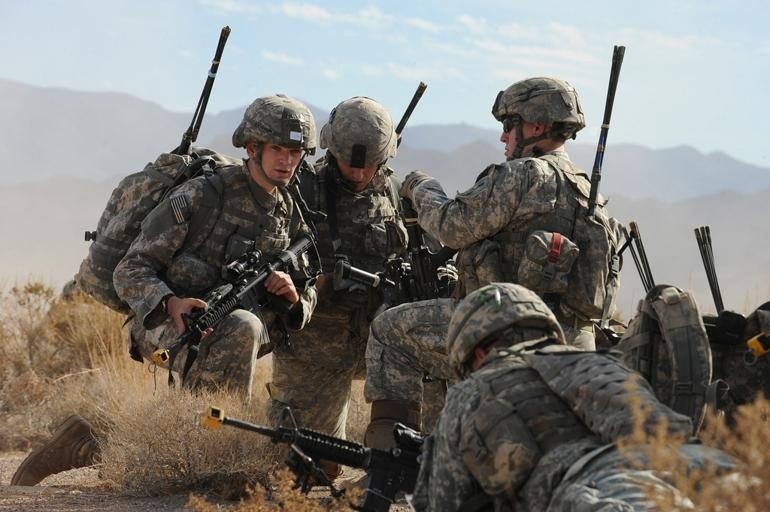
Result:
[334,197,460,306]
[203,406,424,512]
[154,235,322,363]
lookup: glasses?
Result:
[503,116,514,133]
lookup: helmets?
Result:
[320,96,398,169]
[232,94,318,156]
[446,281,566,369]
[492,77,585,132]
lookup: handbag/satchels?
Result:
[567,208,624,319]
[517,230,580,294]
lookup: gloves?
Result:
[399,171,430,200]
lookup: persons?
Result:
[346,76,607,506]
[10,95,326,488]
[265,97,459,486]
[411,282,762,512]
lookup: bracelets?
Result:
[406,176,430,196]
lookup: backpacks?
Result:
[74,147,243,316]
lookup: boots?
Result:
[11,414,102,487]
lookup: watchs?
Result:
[158,295,176,314]
